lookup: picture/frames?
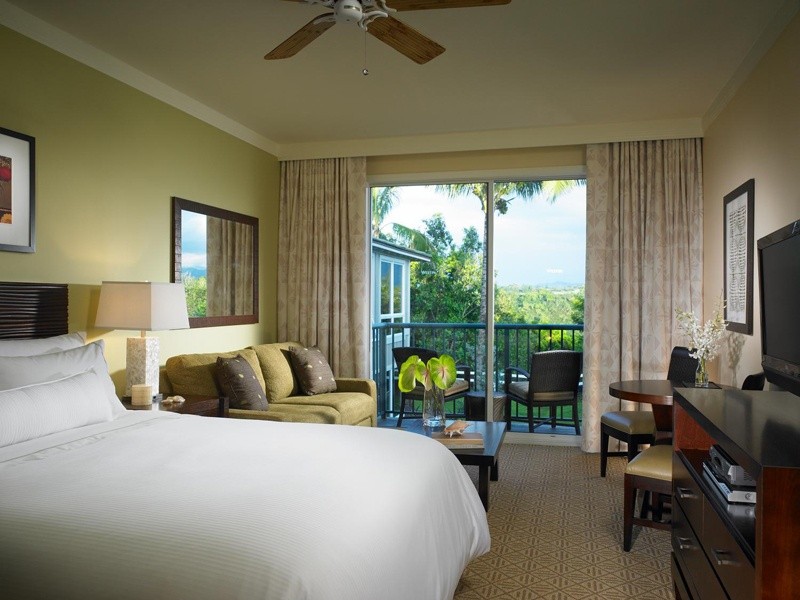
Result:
[723,178,755,336]
[0,126,36,254]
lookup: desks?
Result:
[466,391,507,422]
[408,420,508,512]
[609,380,741,447]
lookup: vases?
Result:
[695,362,709,388]
[423,383,446,427]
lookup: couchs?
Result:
[165,341,377,429]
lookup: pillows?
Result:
[0,339,129,448]
[289,345,337,396]
[217,354,270,411]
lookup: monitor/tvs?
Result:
[756,218,800,398]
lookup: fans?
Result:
[264,0,512,65]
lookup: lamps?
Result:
[94,280,190,402]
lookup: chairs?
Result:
[600,346,765,552]
[504,351,584,435]
[392,347,471,436]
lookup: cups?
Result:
[132,384,152,405]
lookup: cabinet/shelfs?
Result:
[672,386,800,600]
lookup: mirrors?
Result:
[172,196,259,328]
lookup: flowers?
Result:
[674,289,729,386]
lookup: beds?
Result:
[0,280,490,600]
